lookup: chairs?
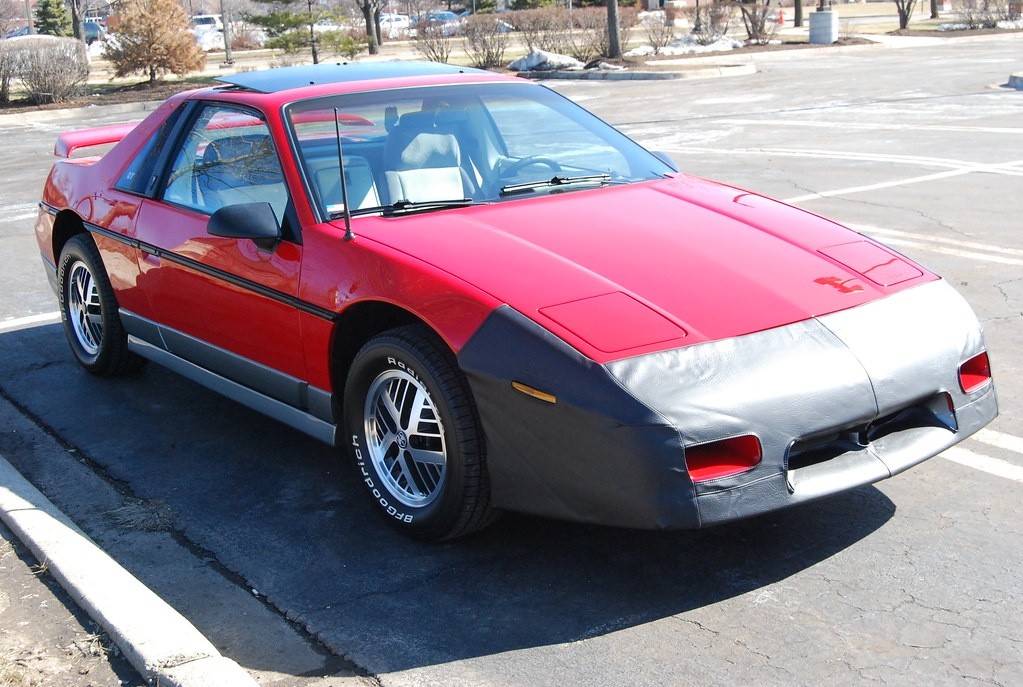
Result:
[192,133,322,226]
[380,110,484,209]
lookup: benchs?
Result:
[301,141,391,216]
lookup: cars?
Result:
[6,8,518,44]
[36,60,997,549]
